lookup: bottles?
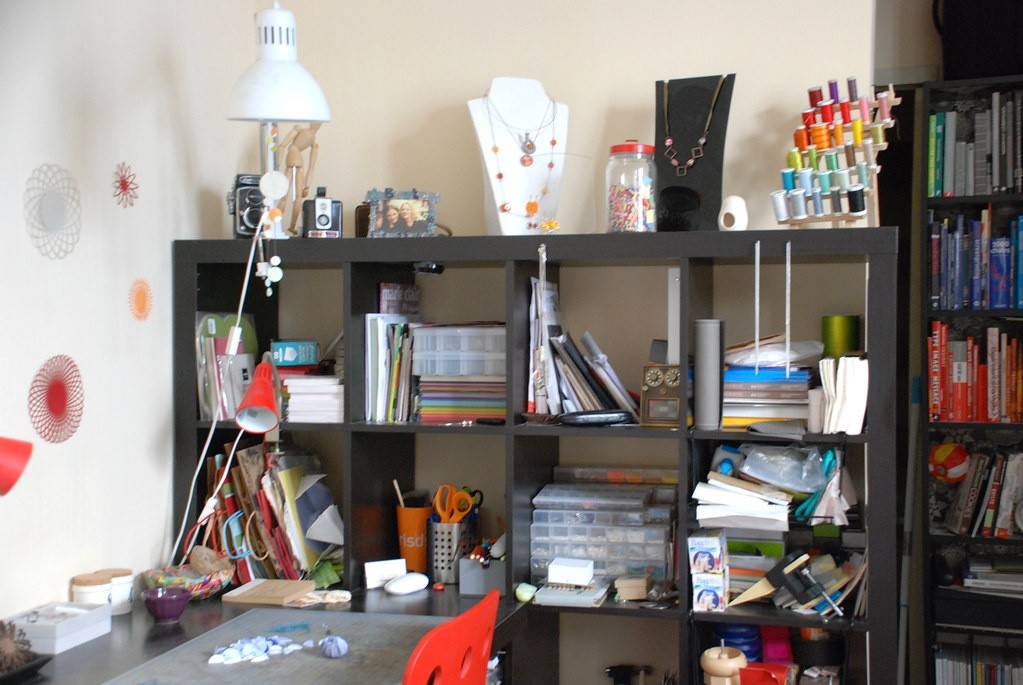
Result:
[605,140,657,233]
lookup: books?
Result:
[532,582,611,608]
[195,314,344,423]
[963,557,1023,591]
[926,206,1023,309]
[729,549,868,620]
[361,283,508,423]
[524,277,641,424]
[947,454,1023,538]
[666,266,681,366]
[927,320,1023,424]
[935,642,1023,685]
[691,469,791,531]
[925,87,1023,198]
[723,332,869,435]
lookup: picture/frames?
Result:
[365,188,439,238]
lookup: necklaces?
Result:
[657,79,723,178]
[483,94,561,238]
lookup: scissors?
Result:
[434,483,473,522]
[461,483,483,521]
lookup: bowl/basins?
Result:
[142,568,236,599]
[142,589,189,624]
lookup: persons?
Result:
[369,192,436,237]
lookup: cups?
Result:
[398,506,431,572]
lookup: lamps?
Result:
[0,436,34,498]
[226,0,331,239]
[178,352,287,565]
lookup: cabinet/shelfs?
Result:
[902,73,1023,685]
[172,224,899,685]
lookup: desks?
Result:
[0,591,529,685]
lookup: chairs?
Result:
[401,589,501,685]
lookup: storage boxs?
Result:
[459,555,507,598]
[411,327,507,376]
[270,338,319,366]
[529,464,676,586]
[547,557,594,586]
[692,565,731,613]
[11,601,112,656]
[687,529,730,574]
[614,574,652,601]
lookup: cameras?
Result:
[301,187,343,237]
[227,174,266,238]
